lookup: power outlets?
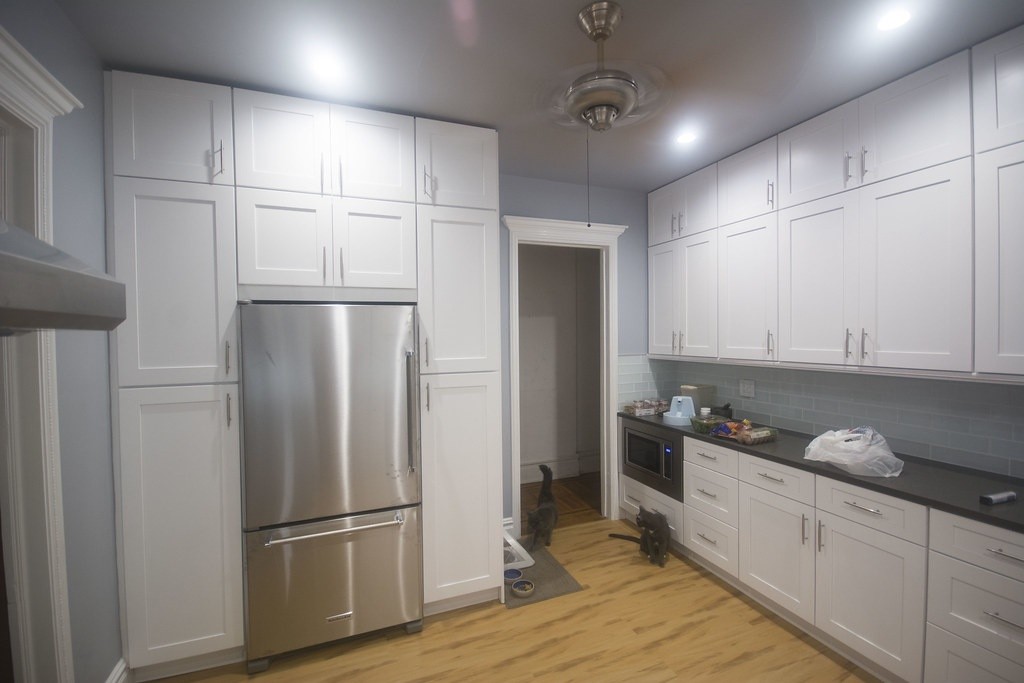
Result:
[740,379,755,398]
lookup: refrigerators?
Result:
[236,300,424,674]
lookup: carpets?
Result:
[505,535,583,612]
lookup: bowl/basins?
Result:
[512,580,534,597]
[690,415,728,434]
[504,568,523,586]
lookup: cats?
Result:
[523,464,557,553]
[609,505,671,568]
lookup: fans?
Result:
[550,2,674,134]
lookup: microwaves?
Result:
[616,414,683,502]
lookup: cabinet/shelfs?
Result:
[718,136,778,225]
[684,437,739,578]
[781,155,976,380]
[647,165,718,247]
[420,205,500,375]
[230,87,418,203]
[422,369,503,609]
[717,212,781,361]
[120,384,246,671]
[105,70,233,185]
[973,26,1024,152]
[105,186,237,388]
[618,473,684,544]
[922,509,1024,683]
[416,118,499,210]
[973,141,1024,386]
[738,452,930,683]
[233,188,418,287]
[647,228,718,362]
[777,50,971,209]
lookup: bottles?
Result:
[701,407,710,416]
